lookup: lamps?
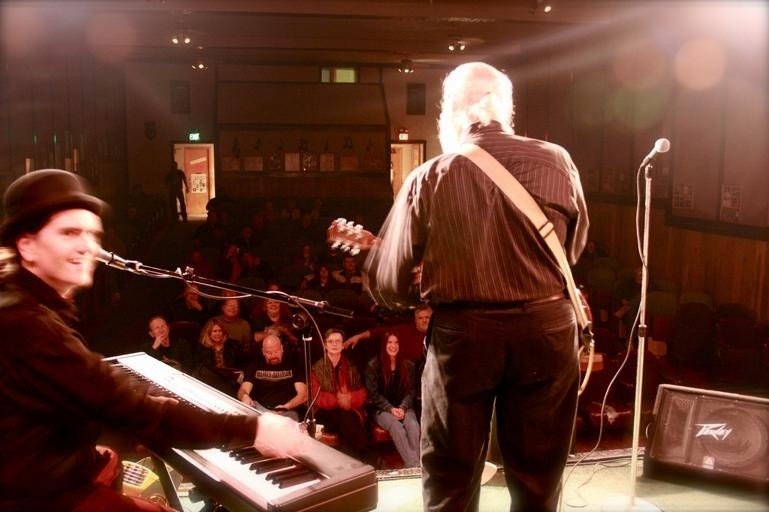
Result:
[168,23,192,48]
[395,57,417,75]
[446,23,473,53]
[189,42,210,73]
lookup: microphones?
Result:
[640,137,670,168]
[92,243,142,277]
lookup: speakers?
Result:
[643,382,769,495]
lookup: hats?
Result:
[0,168,113,246]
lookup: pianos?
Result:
[98,349,378,512]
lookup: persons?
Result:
[164,159,191,220]
[379,62,590,512]
[142,197,651,469]
[1,169,313,512]
